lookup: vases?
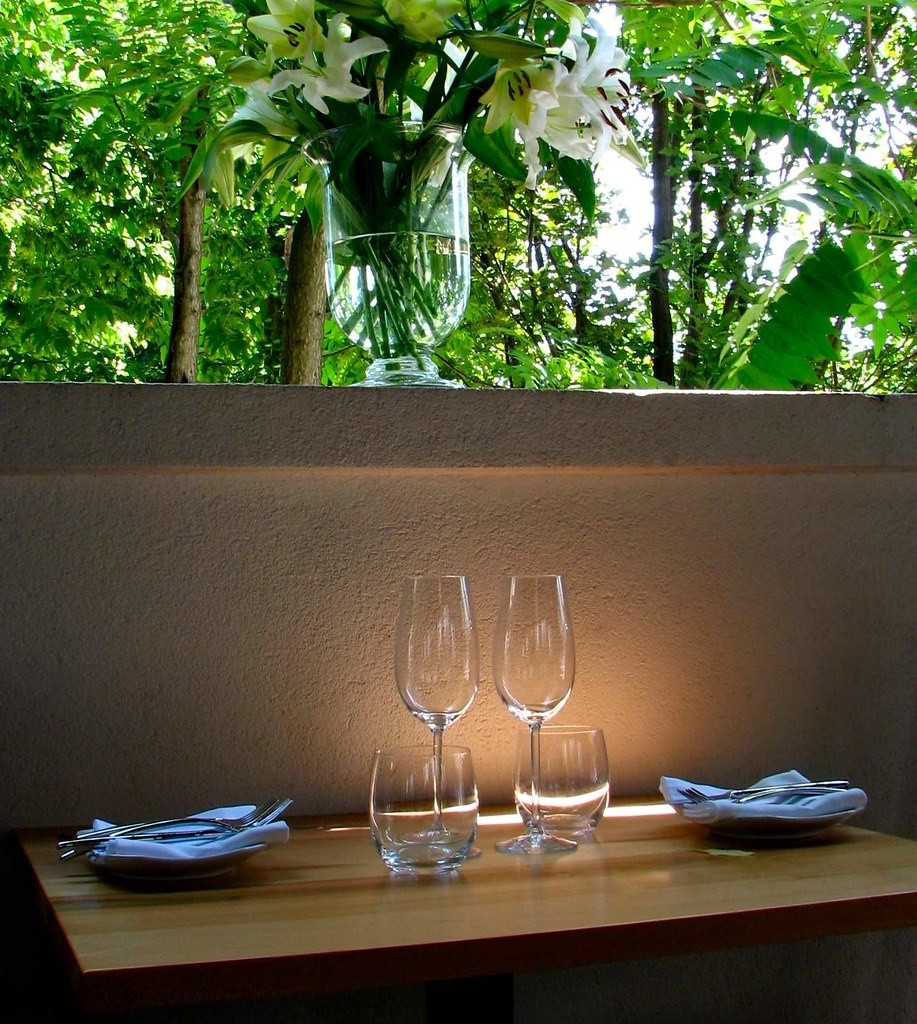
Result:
[300,120,470,390]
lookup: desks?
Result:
[20,794,917,1024]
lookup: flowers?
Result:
[154,0,651,369]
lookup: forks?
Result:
[59,794,279,828]
[53,798,293,859]
[678,787,847,803]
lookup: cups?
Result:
[515,726,610,837]
[368,745,480,873]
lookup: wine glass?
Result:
[492,575,578,855]
[393,575,480,842]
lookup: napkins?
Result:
[78,805,289,862]
[657,771,867,824]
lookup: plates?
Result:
[76,841,268,901]
[674,806,866,841]
